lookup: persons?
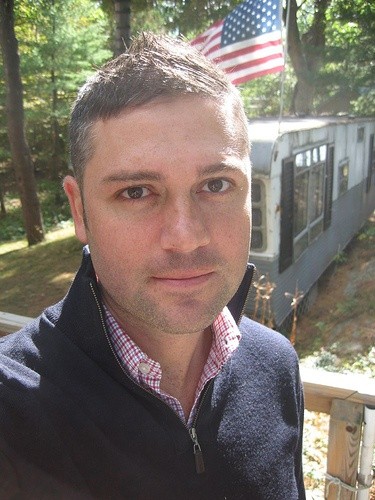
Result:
[0,31,309,500]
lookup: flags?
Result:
[179,0,284,86]
